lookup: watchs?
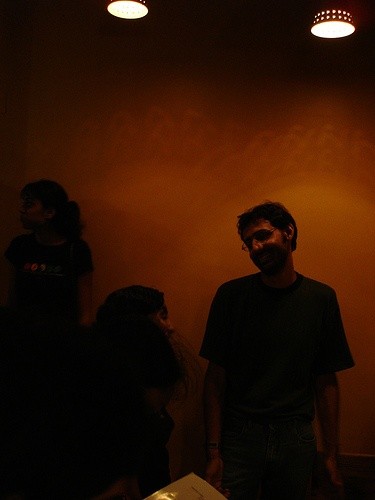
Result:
[208,443,216,448]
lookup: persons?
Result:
[197,202,371,500]
[0,286,175,500]
[4,179,92,327]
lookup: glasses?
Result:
[242,225,280,252]
